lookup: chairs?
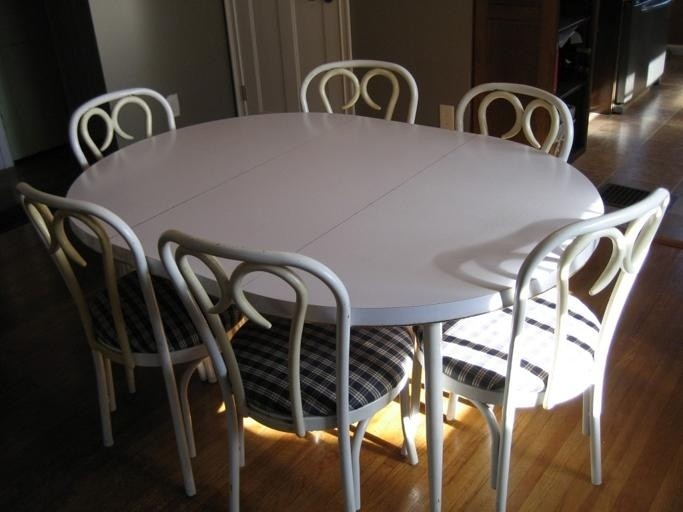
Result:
[452,79,577,165]
[157,225,418,511]
[68,84,220,416]
[298,57,421,125]
[401,185,676,512]
[13,181,261,500]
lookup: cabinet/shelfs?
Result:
[470,0,600,165]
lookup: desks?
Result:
[63,111,608,512]
